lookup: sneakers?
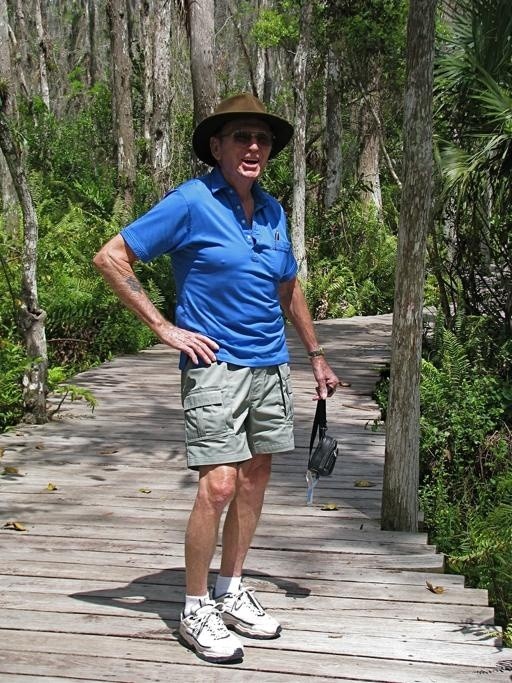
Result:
[179,598,244,663]
[208,583,282,639]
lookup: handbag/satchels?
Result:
[307,384,339,476]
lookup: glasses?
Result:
[220,129,276,147]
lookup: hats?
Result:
[192,93,293,168]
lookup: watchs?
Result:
[307,347,326,360]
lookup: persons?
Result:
[91,93,340,663]
